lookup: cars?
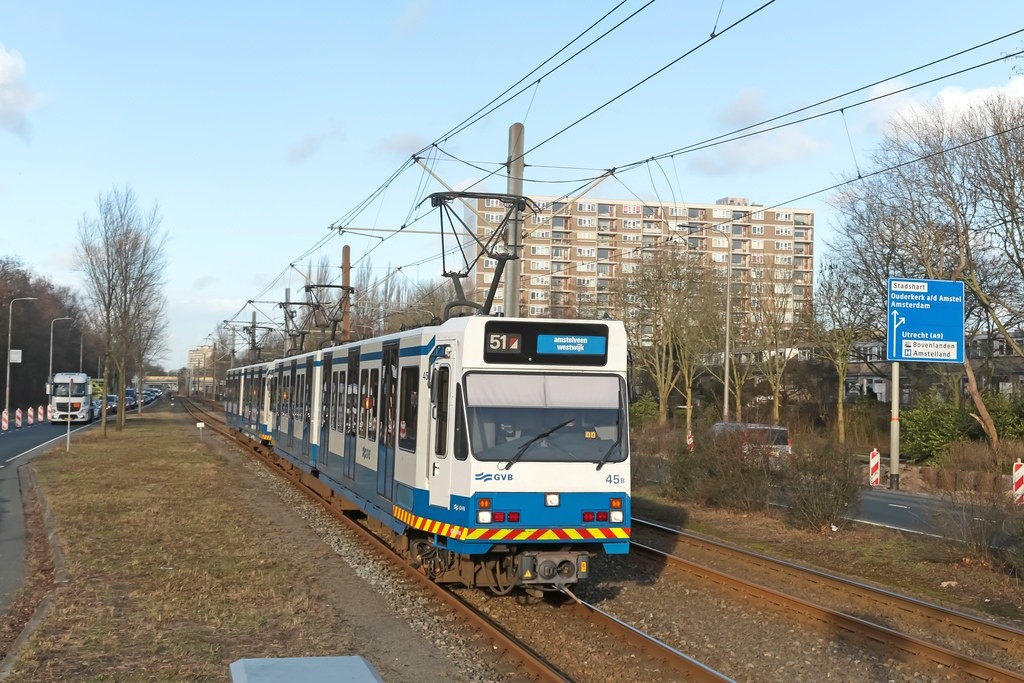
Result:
[100,387,167,415]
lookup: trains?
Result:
[220,318,631,594]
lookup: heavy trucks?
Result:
[45,372,101,423]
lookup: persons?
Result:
[561,409,579,428]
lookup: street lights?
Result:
[678,221,734,421]
[48,317,73,404]
[5,296,38,409]
[78,330,94,373]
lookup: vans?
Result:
[708,419,792,474]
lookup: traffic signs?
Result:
[885,277,964,364]
